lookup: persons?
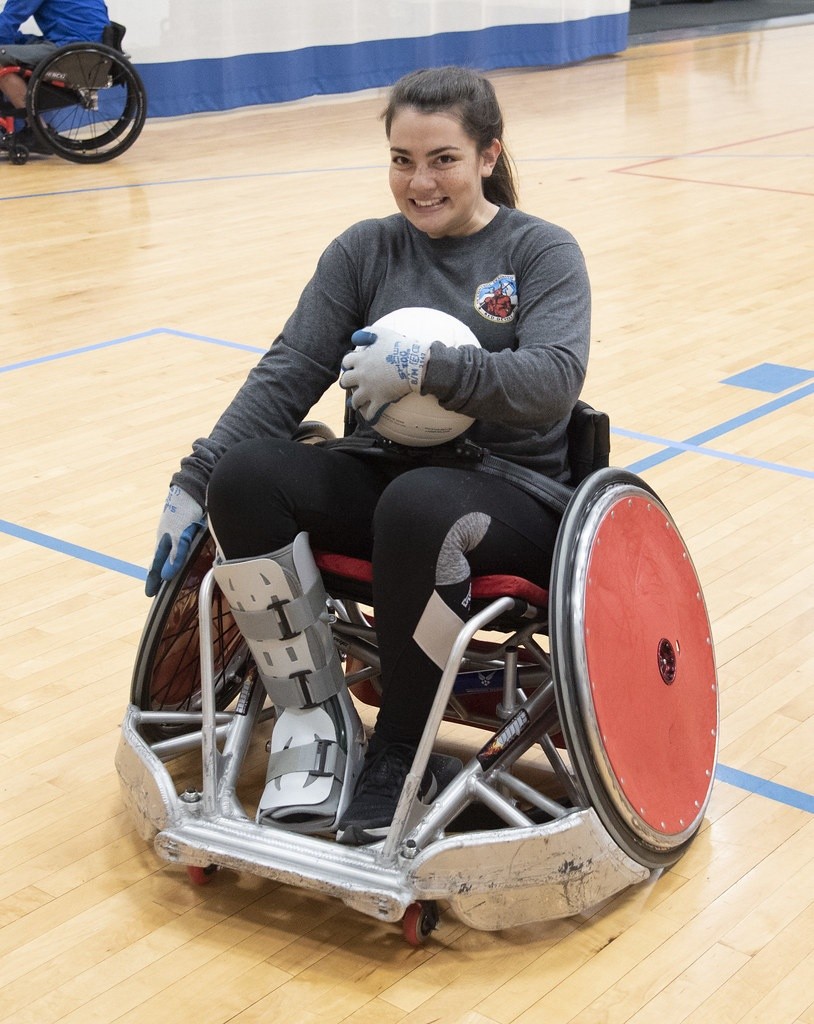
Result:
[0,0,111,142]
[145,64,592,846]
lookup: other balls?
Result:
[338,308,484,445]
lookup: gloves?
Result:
[145,484,205,597]
[339,325,430,427]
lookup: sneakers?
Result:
[336,734,437,846]
[0,123,57,155]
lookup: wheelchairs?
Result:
[2,18,149,167]
[113,398,721,947]
